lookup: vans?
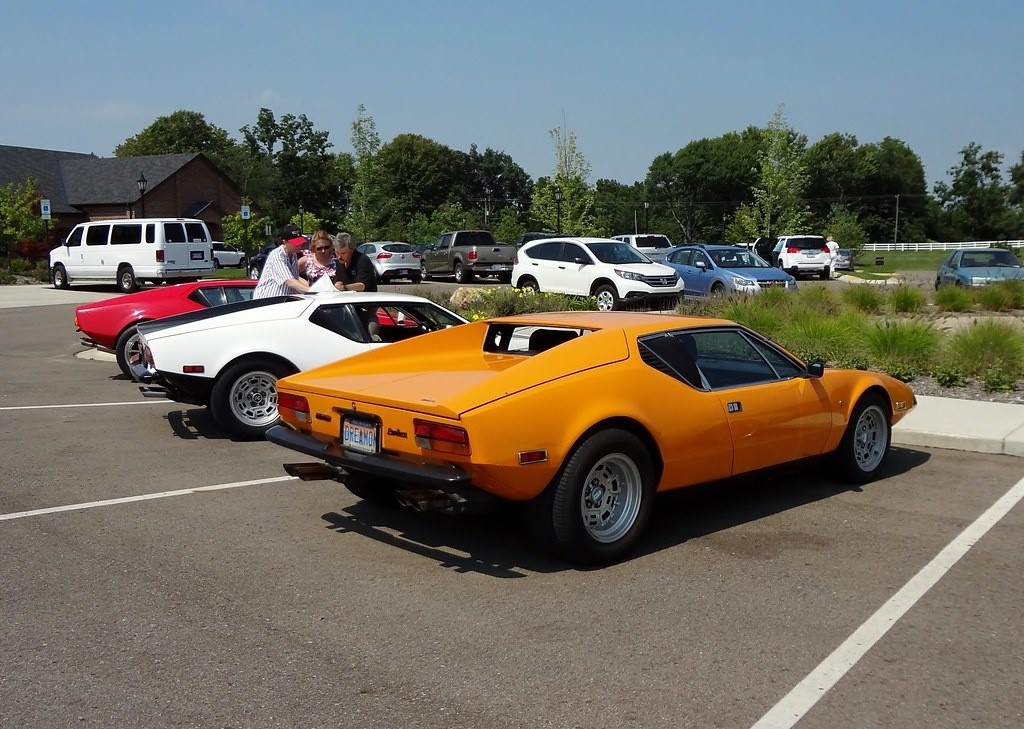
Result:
[514,232,577,251]
[48,217,217,294]
[609,234,674,265]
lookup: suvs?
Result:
[510,237,685,311]
[752,235,831,280]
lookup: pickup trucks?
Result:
[420,230,519,285]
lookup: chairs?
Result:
[724,253,738,266]
[527,329,579,352]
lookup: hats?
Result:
[281,225,307,246]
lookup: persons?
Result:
[333,233,378,292]
[297,231,336,285]
[772,239,784,267]
[826,236,839,279]
[254,226,310,299]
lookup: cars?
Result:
[412,243,430,254]
[832,249,855,271]
[661,242,798,302]
[248,233,313,280]
[211,240,248,269]
[731,242,755,251]
[935,248,1024,294]
[355,242,422,285]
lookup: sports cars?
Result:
[73,279,419,385]
[130,290,591,443]
[264,310,919,568]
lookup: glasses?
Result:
[316,245,333,251]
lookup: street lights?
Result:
[644,197,651,234]
[136,171,147,218]
[554,184,565,233]
[298,199,305,234]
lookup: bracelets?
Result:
[344,284,348,290]
